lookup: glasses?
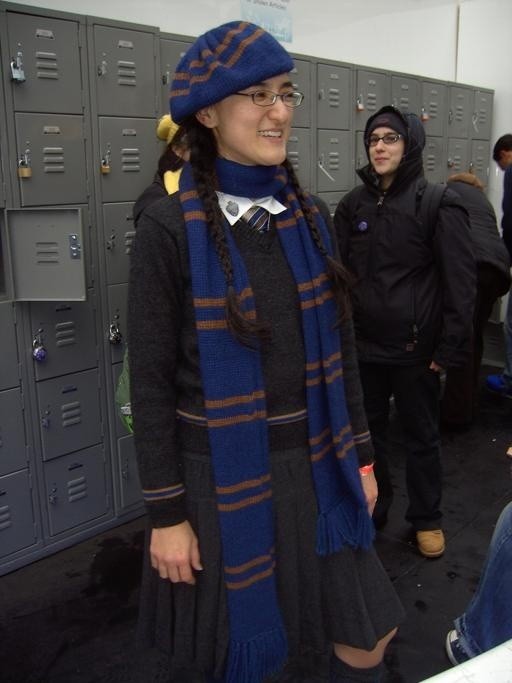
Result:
[364,133,405,146]
[231,87,306,110]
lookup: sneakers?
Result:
[415,529,446,559]
[486,374,512,398]
[446,629,471,666]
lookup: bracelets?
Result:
[359,462,375,476]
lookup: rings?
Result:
[374,497,378,502]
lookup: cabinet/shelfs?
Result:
[0,1,165,579]
[162,33,494,217]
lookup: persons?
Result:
[486,132,512,400]
[435,171,511,434]
[130,113,193,229]
[333,106,479,558]
[444,500,511,665]
[125,21,408,683]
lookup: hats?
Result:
[155,111,181,145]
[168,20,297,127]
[364,111,409,139]
